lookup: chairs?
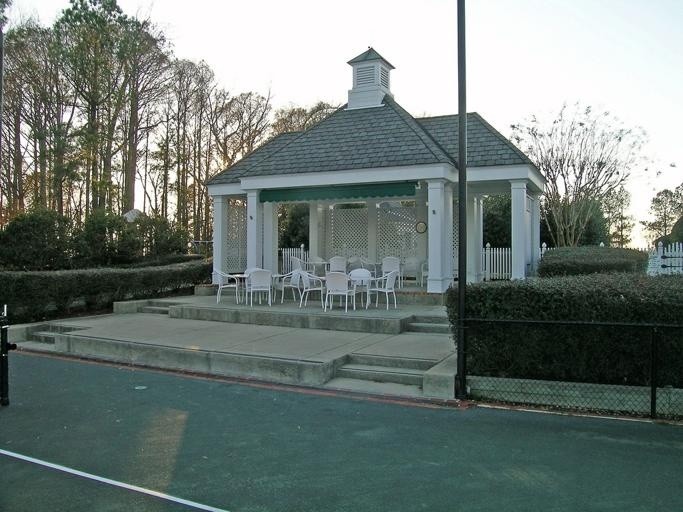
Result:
[214,253,428,310]
